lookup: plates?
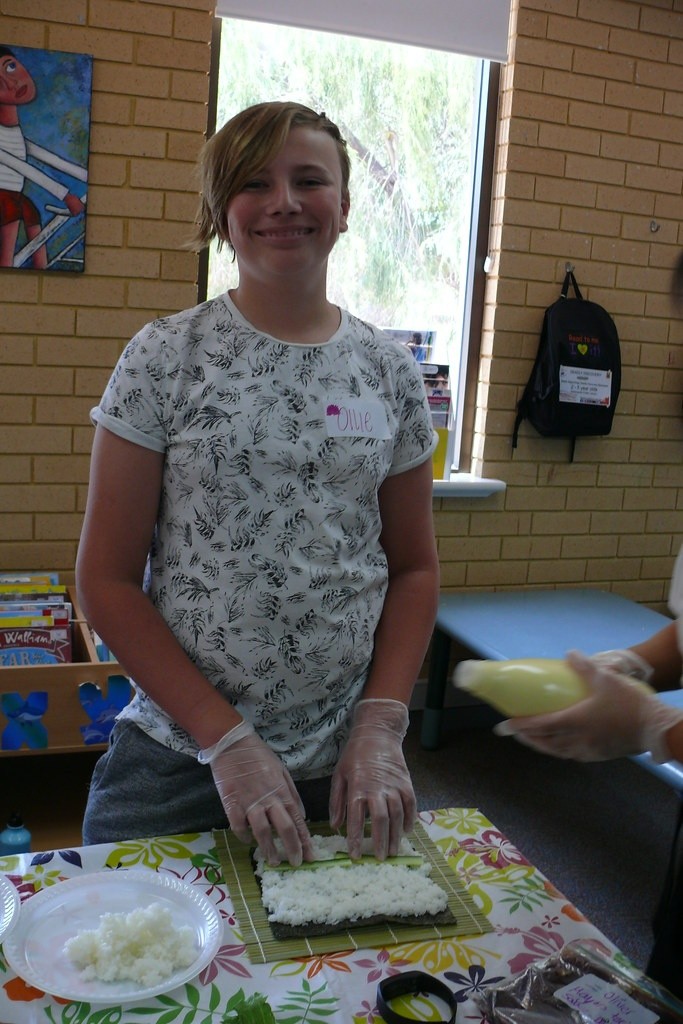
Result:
[0,872,20,945]
[3,869,224,1004]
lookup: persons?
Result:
[74,98,439,873]
[492,253,683,1003]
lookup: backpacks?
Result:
[510,271,620,465]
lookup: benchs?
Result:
[422,587,683,794]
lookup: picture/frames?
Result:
[0,44,95,273]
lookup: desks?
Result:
[0,806,683,1024]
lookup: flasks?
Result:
[0,812,33,857]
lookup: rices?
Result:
[63,835,449,987]
